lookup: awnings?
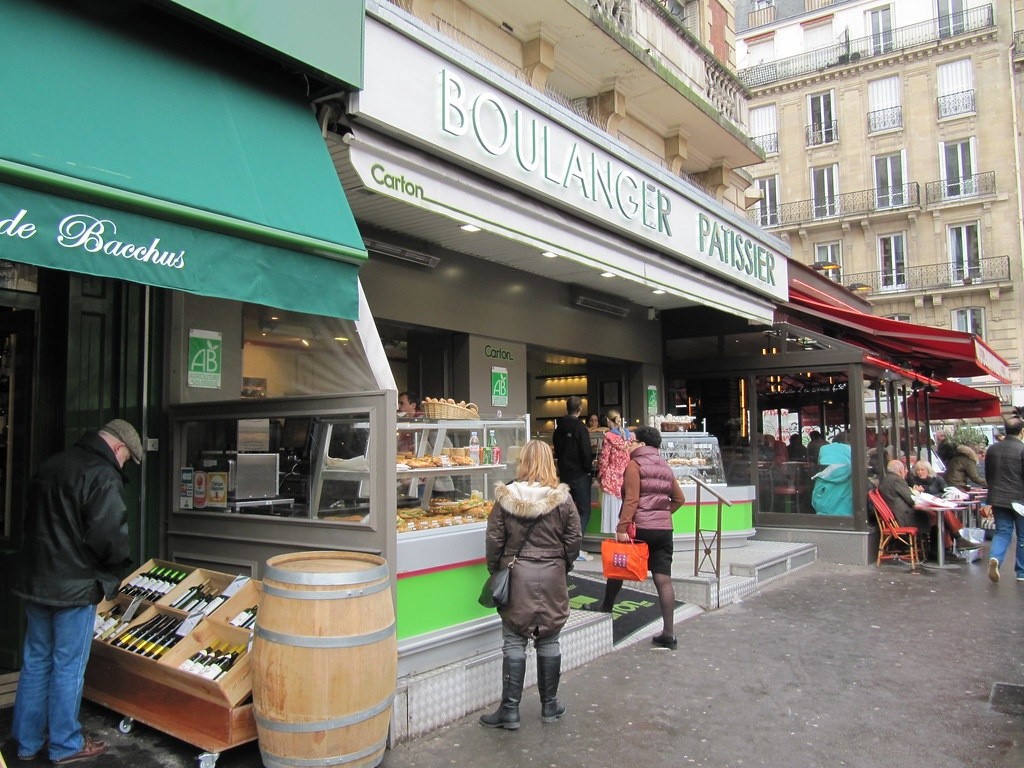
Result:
[0,0,369,321]
[799,399,860,427]
[897,388,1001,421]
[787,294,1012,387]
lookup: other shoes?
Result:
[576,550,593,562]
[583,603,612,613]
[652,635,676,651]
[1016,576,1024,581]
[989,556,1000,583]
[945,554,967,564]
[957,539,983,550]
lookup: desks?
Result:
[948,485,988,563]
[916,507,967,570]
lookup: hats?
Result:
[103,419,142,464]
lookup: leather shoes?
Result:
[54,737,109,766]
[20,736,51,761]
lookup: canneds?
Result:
[478,446,502,465]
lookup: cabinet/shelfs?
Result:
[662,435,727,486]
[310,420,528,532]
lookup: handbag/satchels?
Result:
[601,529,648,582]
[478,568,512,609]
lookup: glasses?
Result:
[628,439,641,444]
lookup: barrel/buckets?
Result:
[251,549,397,768]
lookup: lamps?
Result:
[333,327,349,343]
[255,314,278,338]
[813,262,868,294]
[302,327,317,348]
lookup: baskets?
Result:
[421,401,480,420]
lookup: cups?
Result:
[966,484,971,491]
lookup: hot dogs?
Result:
[395,496,495,532]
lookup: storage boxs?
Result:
[90,556,263,704]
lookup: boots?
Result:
[537,653,566,723]
[479,656,527,729]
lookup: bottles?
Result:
[110,614,184,660]
[177,640,248,683]
[93,604,137,643]
[488,429,497,464]
[119,566,187,602]
[167,577,230,617]
[231,605,259,629]
[468,432,480,468]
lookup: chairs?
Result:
[868,488,928,570]
[770,463,807,514]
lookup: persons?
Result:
[946,445,988,491]
[584,414,608,433]
[283,392,456,502]
[732,427,924,516]
[596,409,635,540]
[583,426,685,651]
[936,429,956,460]
[985,417,1024,582]
[878,460,982,562]
[907,460,948,501]
[479,440,582,729]
[9,419,144,764]
[552,396,594,561]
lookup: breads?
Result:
[396,451,476,468]
[668,457,708,466]
